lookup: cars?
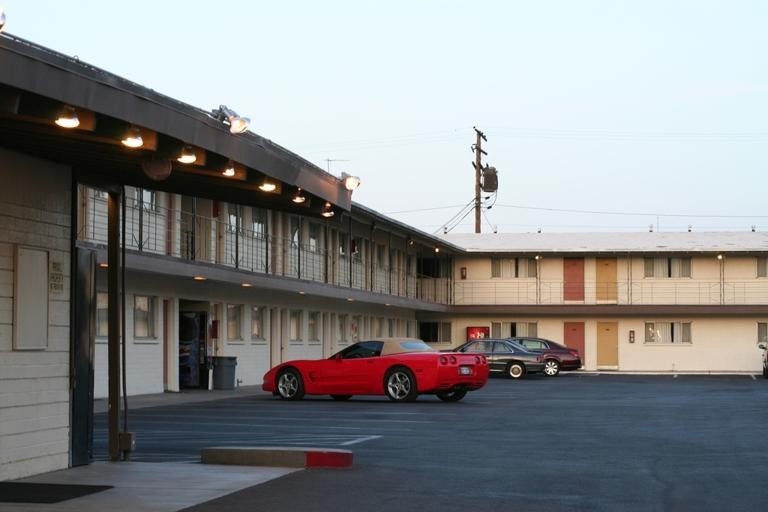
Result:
[439,337,582,380]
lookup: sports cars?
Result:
[261,337,490,403]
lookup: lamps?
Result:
[52,103,335,218]
[341,171,360,191]
